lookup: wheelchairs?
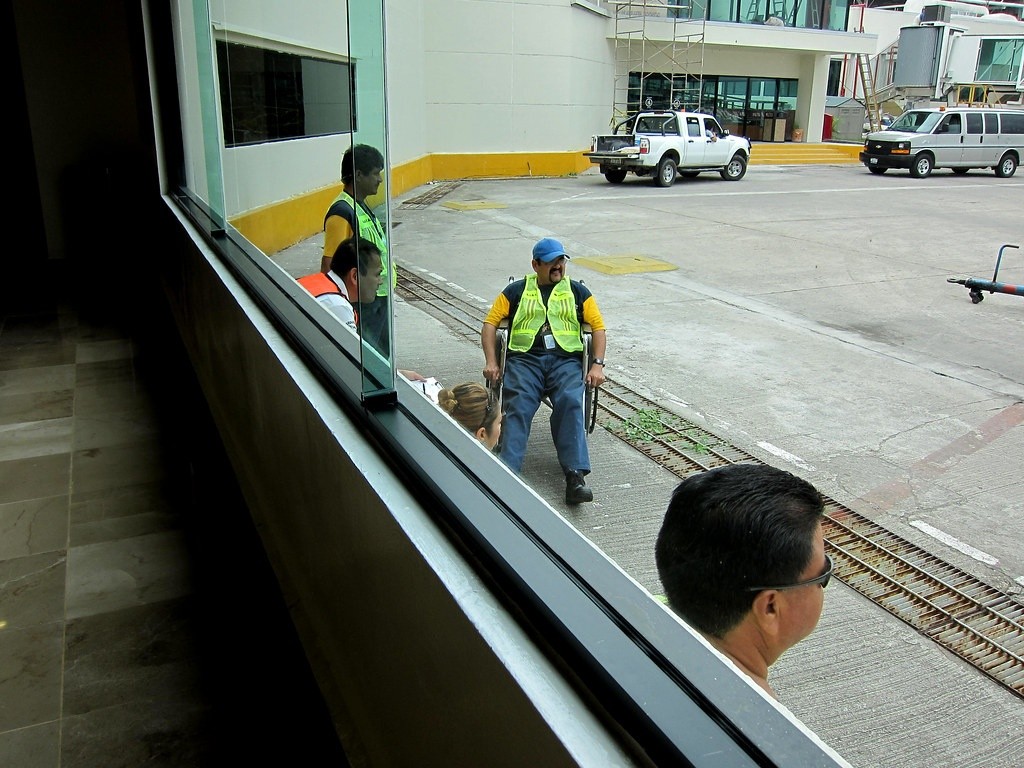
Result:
[487,277,599,438]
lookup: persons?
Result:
[295,237,427,382]
[320,143,397,361]
[481,237,607,505]
[705,121,717,142]
[652,463,827,700]
[437,381,502,451]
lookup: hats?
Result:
[533,237,570,261]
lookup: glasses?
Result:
[538,259,568,265]
[748,554,834,598]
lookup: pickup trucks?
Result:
[583,108,752,186]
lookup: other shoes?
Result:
[565,469,593,507]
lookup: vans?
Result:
[858,106,1024,178]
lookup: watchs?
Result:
[593,358,605,367]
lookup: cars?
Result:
[862,113,891,133]
